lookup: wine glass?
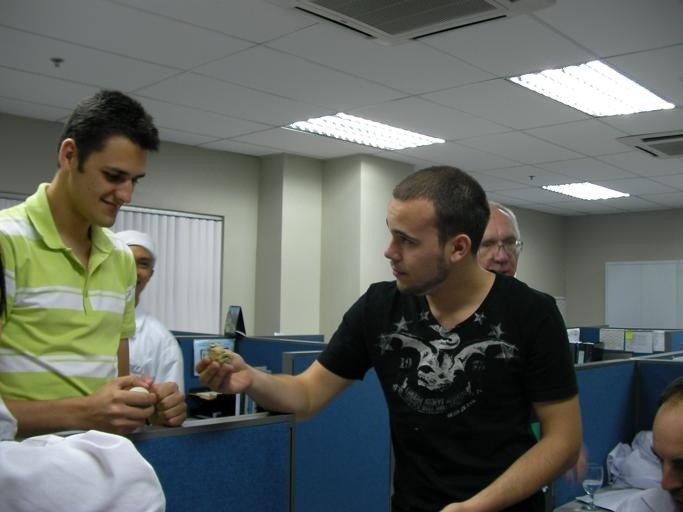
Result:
[578,464,604,512]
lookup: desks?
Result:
[552,477,643,512]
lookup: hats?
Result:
[114,228,158,256]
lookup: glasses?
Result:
[480,236,525,258]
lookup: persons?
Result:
[477,200,521,278]
[196,166,583,512]
[0,253,166,512]
[116,230,185,394]
[0,89,188,436]
[616,375,683,512]
